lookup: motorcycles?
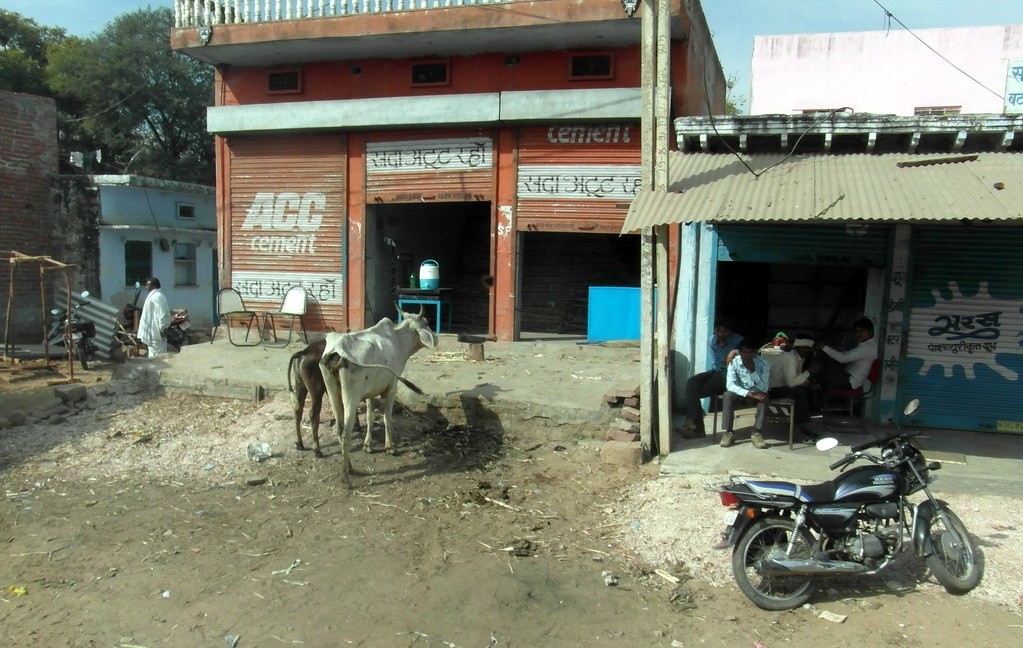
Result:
[123,282,190,345]
[51,291,99,370]
[702,399,981,611]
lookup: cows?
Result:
[286,299,439,490]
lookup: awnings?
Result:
[619,147,1023,237]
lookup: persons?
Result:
[808,318,877,391]
[769,335,819,445]
[136,278,172,358]
[674,316,743,439]
[719,339,769,448]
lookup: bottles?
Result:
[410,273,416,289]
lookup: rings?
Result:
[761,397,764,399]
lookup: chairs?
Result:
[761,350,794,447]
[261,285,309,348]
[822,358,884,435]
[211,288,259,346]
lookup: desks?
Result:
[398,296,452,334]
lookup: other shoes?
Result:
[802,428,818,438]
[682,430,705,438]
[676,425,696,433]
[795,434,817,445]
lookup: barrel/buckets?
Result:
[419,259,440,290]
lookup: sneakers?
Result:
[751,432,767,449]
[720,432,735,447]
